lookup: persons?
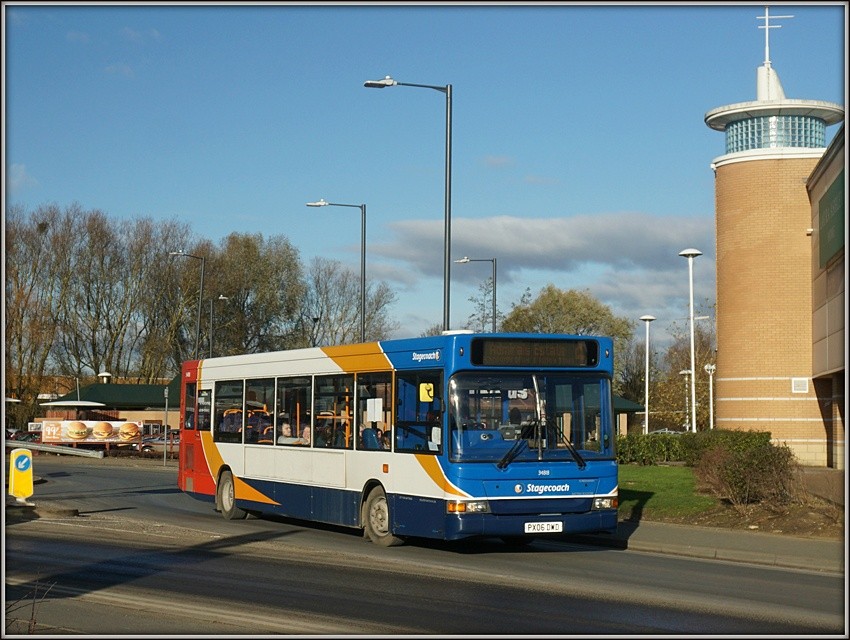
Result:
[273,420,392,450]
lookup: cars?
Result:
[132,429,179,454]
[6,428,120,457]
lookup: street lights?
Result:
[704,364,717,429]
[97,372,112,384]
[209,294,230,358]
[680,369,692,431]
[168,248,206,359]
[454,256,496,333]
[639,315,657,435]
[306,199,366,343]
[362,75,454,331]
[679,248,704,433]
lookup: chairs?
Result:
[223,409,380,449]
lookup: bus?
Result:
[176,329,619,546]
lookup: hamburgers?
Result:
[66,422,88,438]
[119,423,141,440]
[93,422,113,438]
